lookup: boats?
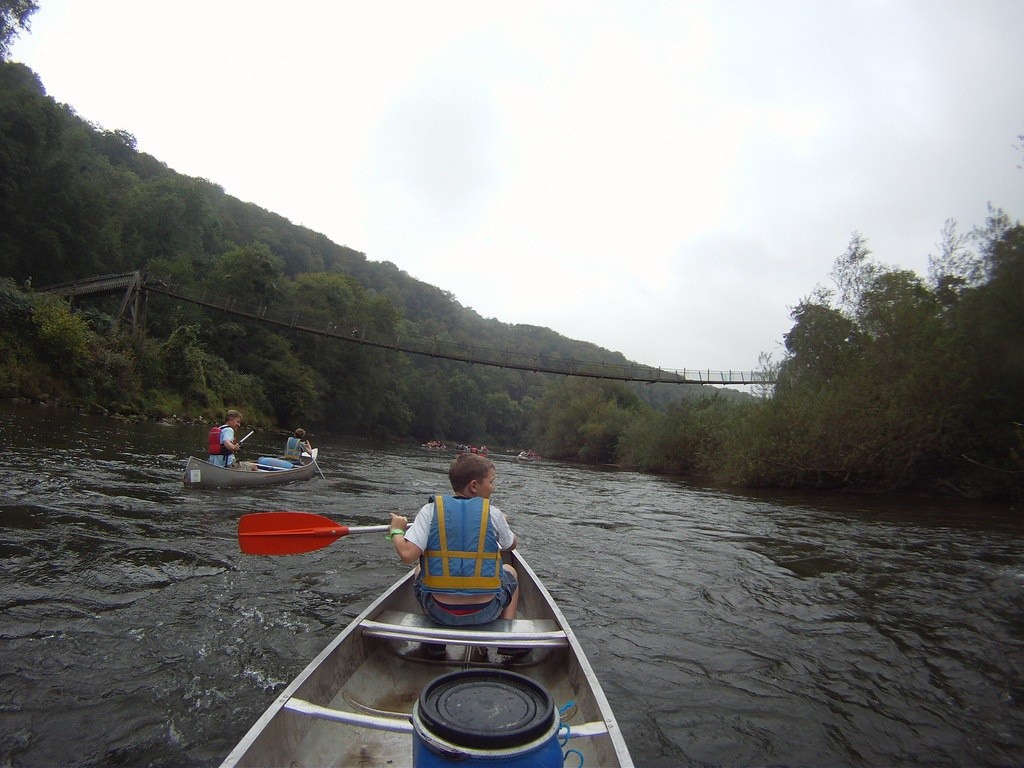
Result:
[184,448,319,488]
[518,452,535,462]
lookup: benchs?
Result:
[361,610,569,648]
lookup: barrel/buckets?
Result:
[408,668,584,768]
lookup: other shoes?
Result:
[423,643,446,655]
[497,647,532,655]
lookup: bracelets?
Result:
[386,529,405,541]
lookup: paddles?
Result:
[237,508,414,554]
[310,449,326,479]
[238,430,254,445]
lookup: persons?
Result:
[520,449,535,457]
[283,429,312,462]
[210,410,242,466]
[390,453,518,626]
[470,446,478,453]
[427,441,444,447]
